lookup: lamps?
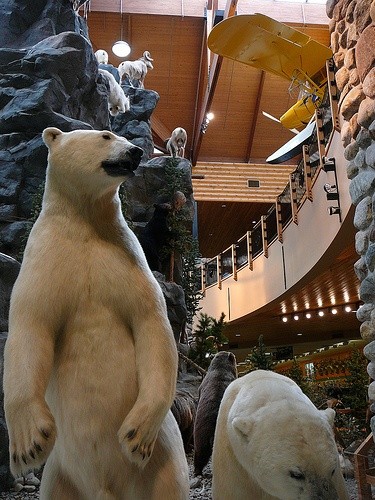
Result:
[320,156,339,215]
[111,41,132,59]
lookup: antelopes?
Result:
[118,50,154,89]
[164,127,187,157]
[97,68,131,117]
[95,49,108,66]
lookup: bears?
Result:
[139,203,174,272]
[192,352,239,478]
